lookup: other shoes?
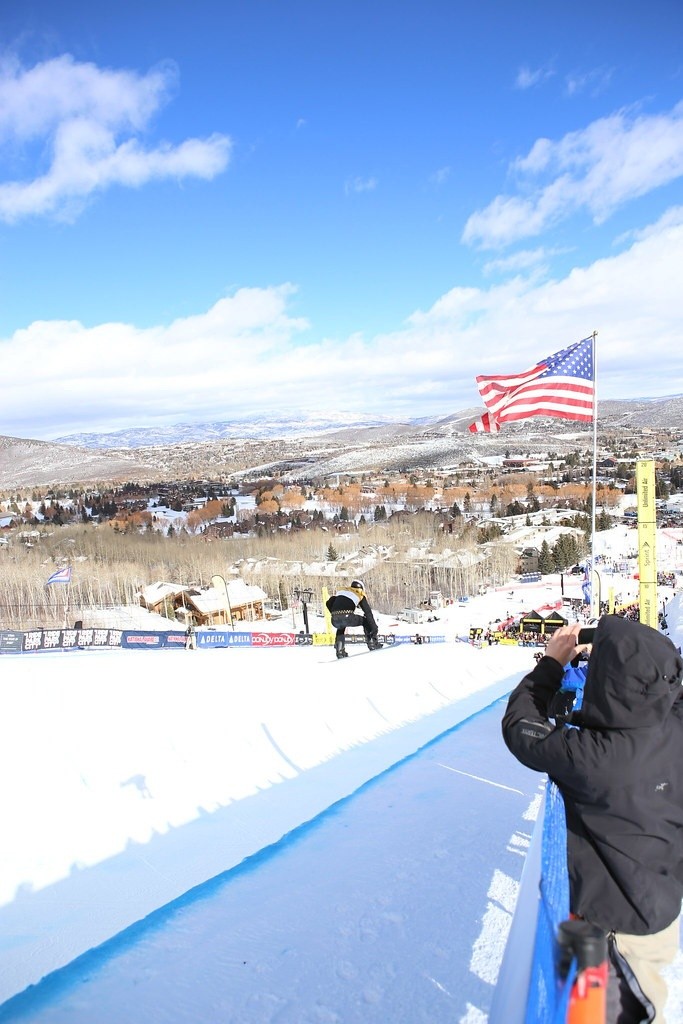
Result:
[368,643,382,650]
[336,651,348,658]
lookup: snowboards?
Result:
[317,641,403,663]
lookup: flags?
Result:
[46,567,70,584]
[469,336,594,433]
[581,563,591,604]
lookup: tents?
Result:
[519,610,568,634]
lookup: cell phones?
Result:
[576,625,597,645]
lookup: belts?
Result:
[332,610,353,615]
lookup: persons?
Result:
[571,601,609,620]
[486,629,494,645]
[657,571,675,588]
[659,613,669,635]
[501,612,683,1024]
[618,603,640,621]
[325,581,383,659]
[504,628,546,643]
[560,643,593,711]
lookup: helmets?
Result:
[351,580,364,590]
